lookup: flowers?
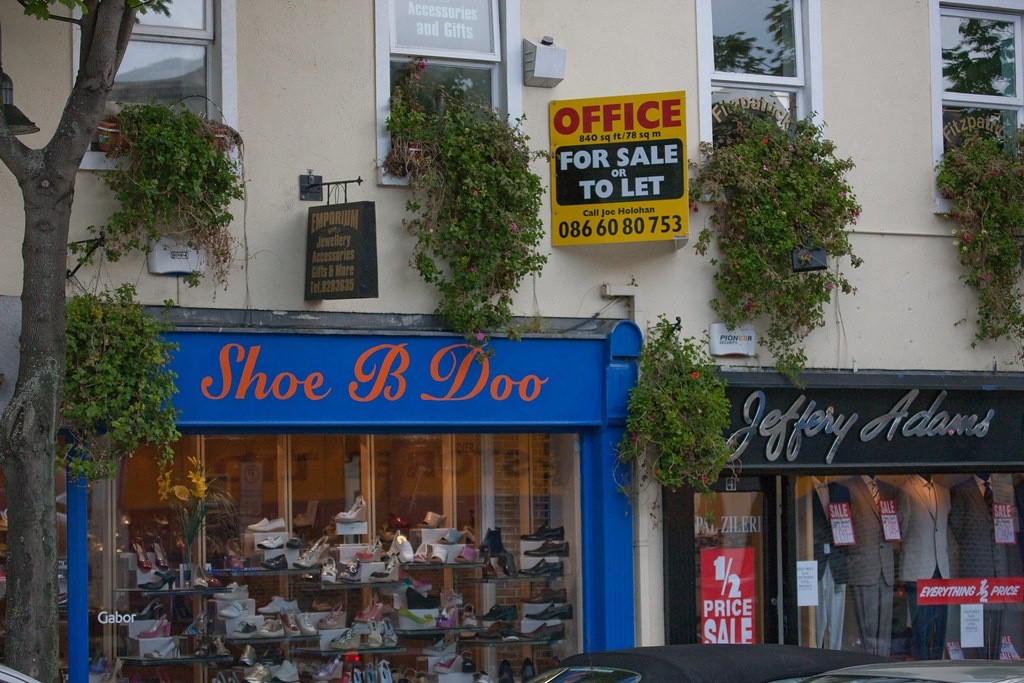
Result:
[156,456,234,562]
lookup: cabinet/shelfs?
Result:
[109,522,572,683]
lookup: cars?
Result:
[523,642,895,683]
[800,659,1024,683]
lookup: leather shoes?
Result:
[477,522,574,683]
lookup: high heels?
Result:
[88,535,230,683]
[292,496,479,683]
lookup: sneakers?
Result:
[219,516,316,683]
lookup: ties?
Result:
[818,483,830,521]
[983,481,993,517]
[870,480,881,516]
[926,483,936,518]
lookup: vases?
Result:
[179,563,196,590]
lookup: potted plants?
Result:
[66,100,253,301]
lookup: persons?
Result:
[811,473,1024,661]
